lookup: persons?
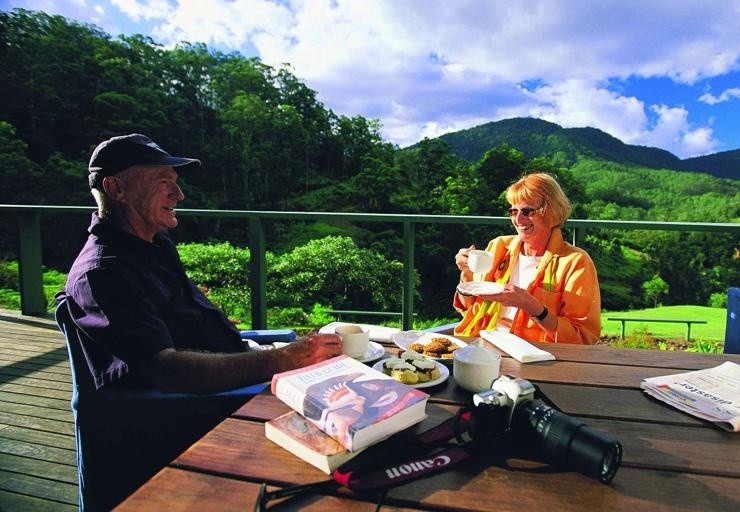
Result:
[450,171,601,344]
[62,132,342,485]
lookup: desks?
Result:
[110,331,740,512]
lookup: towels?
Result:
[480,326,555,365]
[318,322,401,343]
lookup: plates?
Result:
[371,357,451,389]
[392,329,467,362]
[352,341,385,362]
[456,279,507,297]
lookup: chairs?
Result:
[54,290,296,512]
[426,322,460,334]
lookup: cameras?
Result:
[454,376,623,483]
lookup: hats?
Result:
[88,133,201,177]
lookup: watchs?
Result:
[529,304,549,325]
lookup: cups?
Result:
[464,250,495,274]
[334,325,370,357]
[454,348,503,392]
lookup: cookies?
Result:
[409,338,461,358]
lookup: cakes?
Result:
[383,350,440,384]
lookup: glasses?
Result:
[508,203,548,217]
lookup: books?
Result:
[269,353,432,454]
[264,410,393,475]
